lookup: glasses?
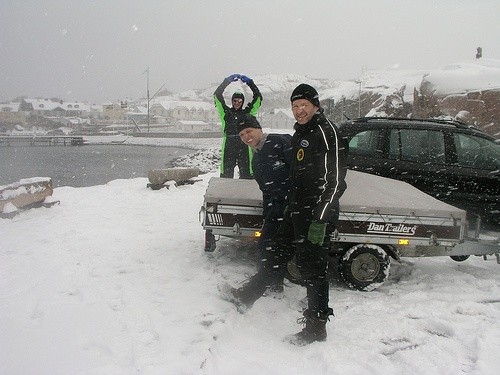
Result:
[234,100,242,103]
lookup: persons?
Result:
[217,83,348,346]
[212,74,263,178]
[235,114,309,312]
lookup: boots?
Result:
[288,309,329,345]
[218,273,268,313]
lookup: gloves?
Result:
[227,74,239,83]
[240,75,250,83]
[307,222,327,246]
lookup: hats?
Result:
[232,92,244,109]
[290,84,320,101]
[236,114,263,134]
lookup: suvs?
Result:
[337,114,500,234]
